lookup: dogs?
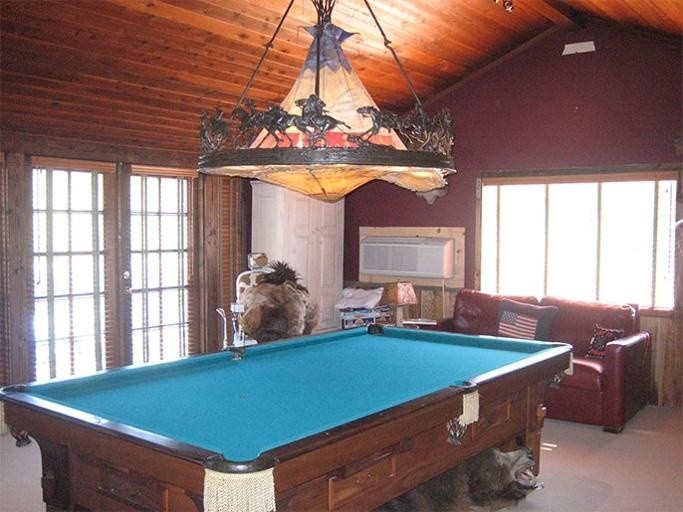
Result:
[371,445,545,511]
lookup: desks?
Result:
[0,323,567,512]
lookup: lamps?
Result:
[194,3,461,207]
[377,280,418,328]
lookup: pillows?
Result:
[493,294,560,349]
[584,323,625,363]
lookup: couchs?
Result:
[424,286,658,435]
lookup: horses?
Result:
[198,107,230,151]
[279,111,353,148]
[412,103,455,155]
[356,104,414,147]
[231,106,296,148]
